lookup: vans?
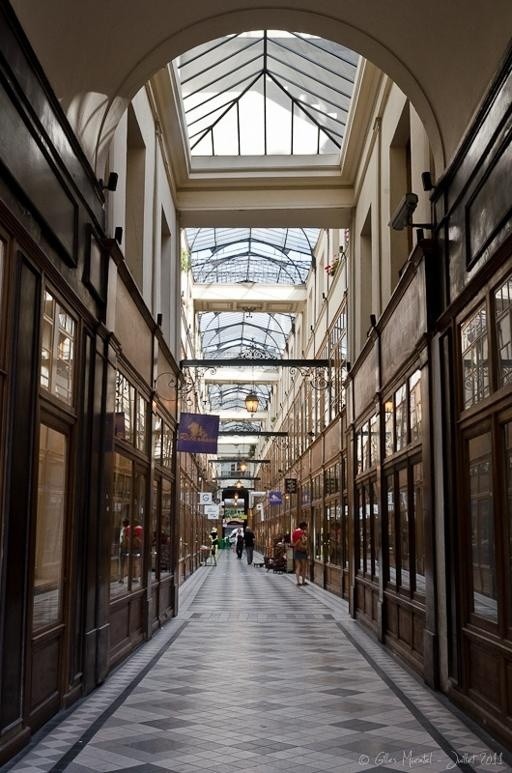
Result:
[225,528,244,544]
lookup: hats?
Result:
[211,527,217,533]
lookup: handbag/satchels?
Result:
[295,536,308,555]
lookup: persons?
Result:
[118,518,131,583]
[329,522,347,565]
[290,520,311,586]
[242,525,256,565]
[209,526,219,558]
[237,527,245,558]
[133,519,144,582]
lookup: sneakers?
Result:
[297,582,309,586]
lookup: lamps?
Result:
[114,226,123,243]
[245,336,259,413]
[422,172,437,191]
[370,314,376,328]
[233,423,247,509]
[100,172,118,191]
[157,313,162,326]
[347,362,351,371]
